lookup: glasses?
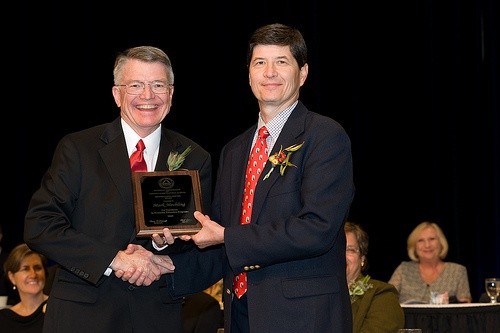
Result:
[117,82,169,95]
[346,246,360,255]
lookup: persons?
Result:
[0,244,49,333]
[343,223,405,333]
[387,222,472,303]
[25,46,215,333]
[114,23,355,333]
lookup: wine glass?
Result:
[484,277,500,303]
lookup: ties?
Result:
[129,139,148,172]
[232,126,270,299]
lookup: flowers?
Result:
[263,141,304,180]
[348,275,372,303]
[167,145,196,172]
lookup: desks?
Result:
[401,298,500,333]
